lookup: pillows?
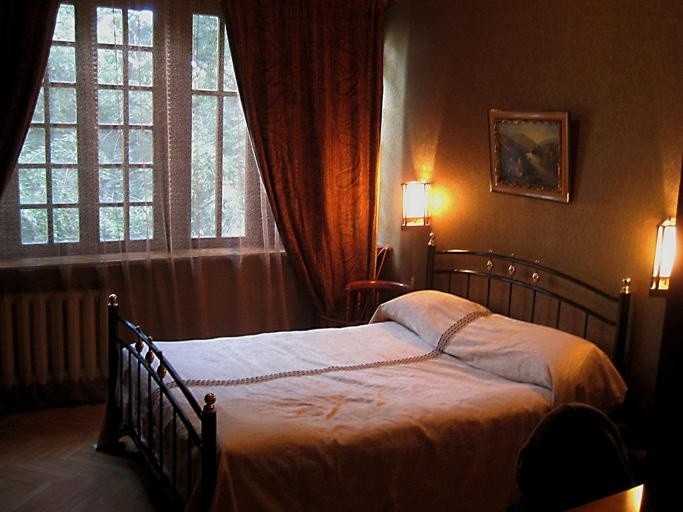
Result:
[369,289,628,408]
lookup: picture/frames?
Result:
[487,107,573,204]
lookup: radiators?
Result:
[0,288,113,390]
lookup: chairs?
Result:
[343,280,413,326]
[513,402,638,512]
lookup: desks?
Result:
[563,481,648,512]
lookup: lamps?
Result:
[648,216,677,298]
[400,181,432,230]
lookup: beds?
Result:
[106,231,632,512]
